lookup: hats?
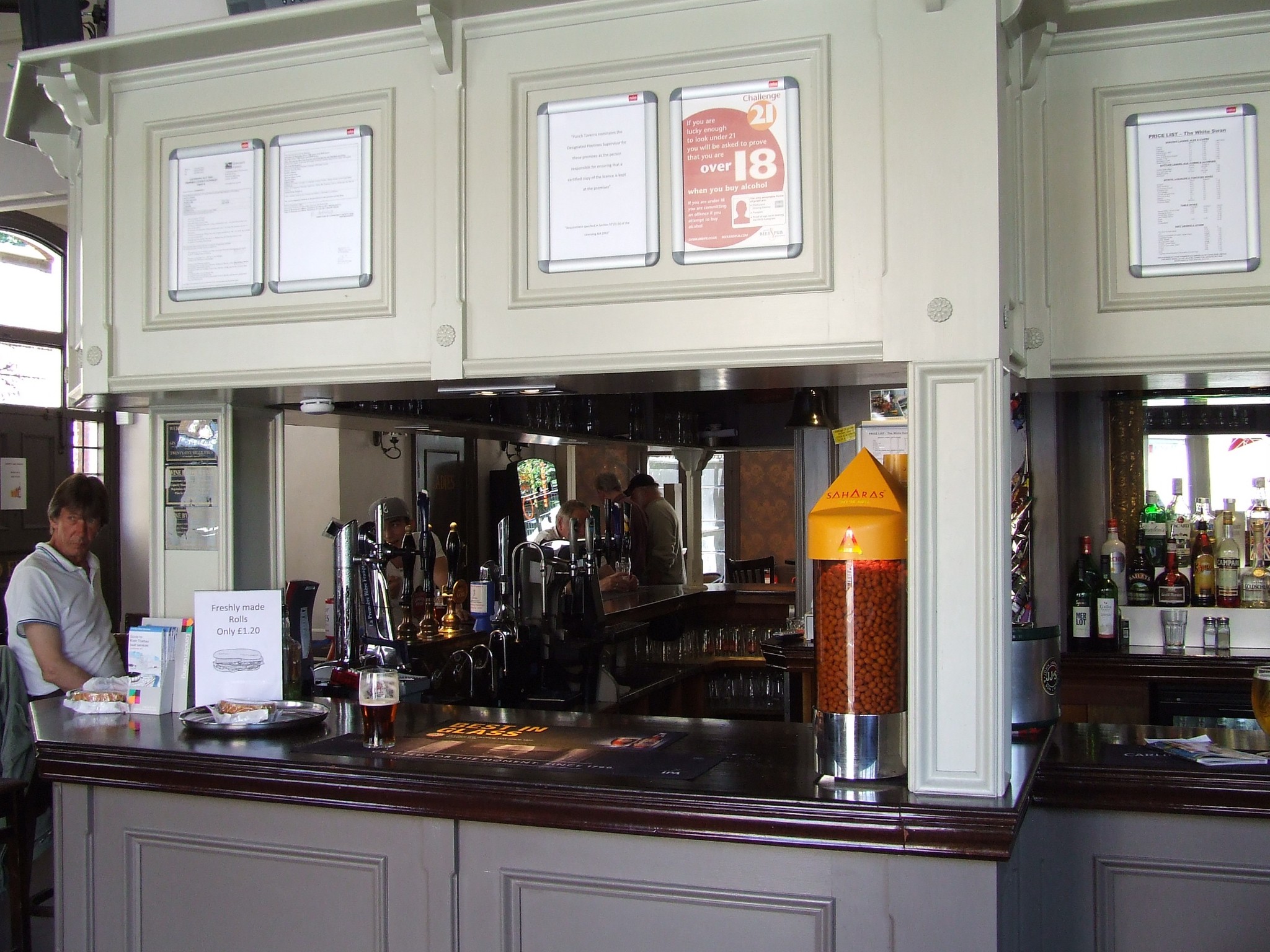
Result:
[623,474,659,496]
[368,495,410,521]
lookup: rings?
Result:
[616,582,618,586]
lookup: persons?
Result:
[535,499,638,594]
[594,472,647,577]
[369,497,448,624]
[622,474,687,585]
[4,472,127,703]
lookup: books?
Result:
[1152,740,1268,766]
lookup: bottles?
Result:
[1245,478,1256,567]
[1072,535,1101,594]
[1189,497,1216,557]
[1139,490,1167,567]
[1240,523,1270,609]
[1101,519,1128,606]
[1190,521,1216,608]
[1216,498,1247,569]
[1216,616,1230,650]
[1166,478,1192,568]
[1154,537,1190,608]
[1066,560,1092,652]
[1215,511,1241,608]
[1125,529,1155,606]
[1092,554,1118,652]
[1250,477,1270,567]
[1204,616,1216,649]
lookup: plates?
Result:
[772,630,804,640]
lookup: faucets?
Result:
[604,500,633,576]
[568,517,580,590]
[584,516,596,583]
[589,503,603,568]
[511,541,547,632]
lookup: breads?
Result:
[219,698,276,715]
[72,689,127,701]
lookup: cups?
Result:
[785,616,805,630]
[630,622,788,706]
[1251,665,1270,736]
[1160,609,1188,651]
[615,557,631,592]
[358,667,399,749]
